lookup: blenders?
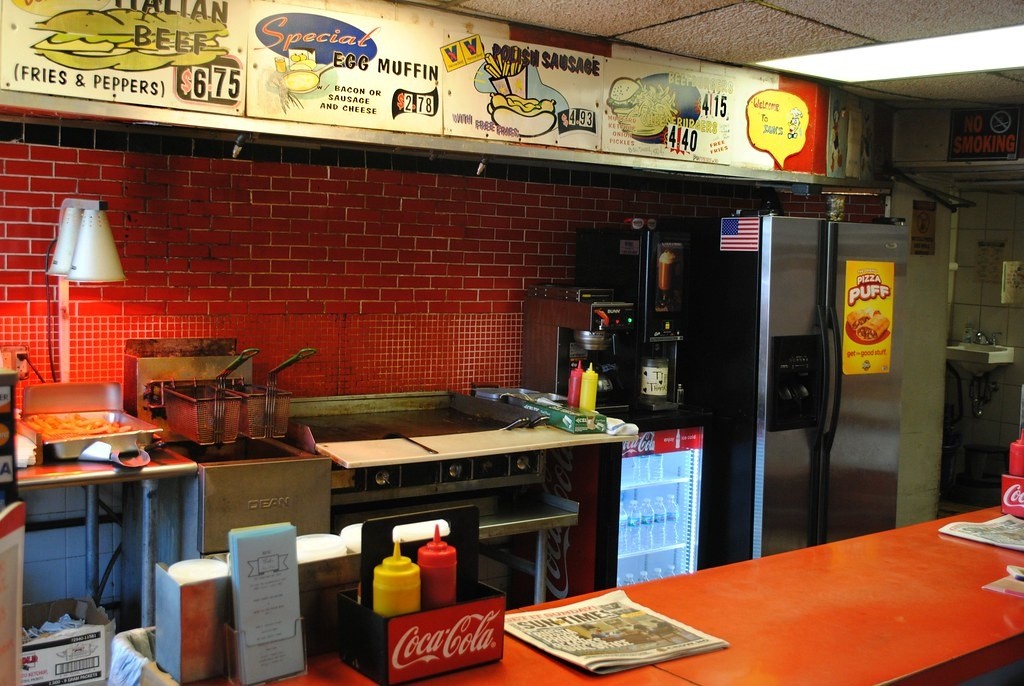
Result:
[521,282,635,414]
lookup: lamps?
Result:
[45,207,81,277]
[66,208,128,282]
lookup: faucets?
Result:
[975,332,990,345]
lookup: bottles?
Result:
[568,360,584,407]
[1009,427,1024,478]
[578,363,599,411]
[417,524,458,611]
[372,539,421,618]
[615,454,679,587]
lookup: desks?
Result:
[176,505,1024,685]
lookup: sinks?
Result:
[945,341,1015,376]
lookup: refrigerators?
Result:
[572,213,910,572]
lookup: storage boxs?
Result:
[22,596,112,686]
[335,584,507,685]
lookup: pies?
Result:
[847,310,891,341]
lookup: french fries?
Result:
[26,414,133,435]
